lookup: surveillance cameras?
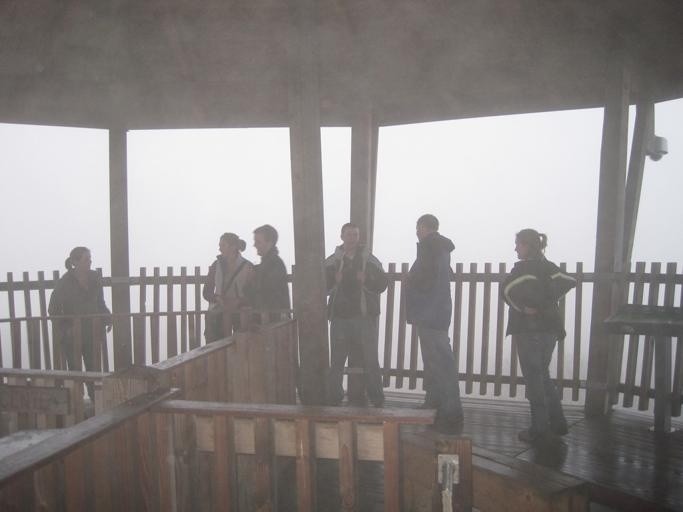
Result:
[647,134,669,161]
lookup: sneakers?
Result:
[518,420,568,442]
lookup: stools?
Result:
[377,415,681,512]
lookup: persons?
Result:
[400,212,467,437]
[249,224,300,391]
[47,246,113,402]
[200,232,257,343]
[496,228,578,446]
[322,222,391,409]
